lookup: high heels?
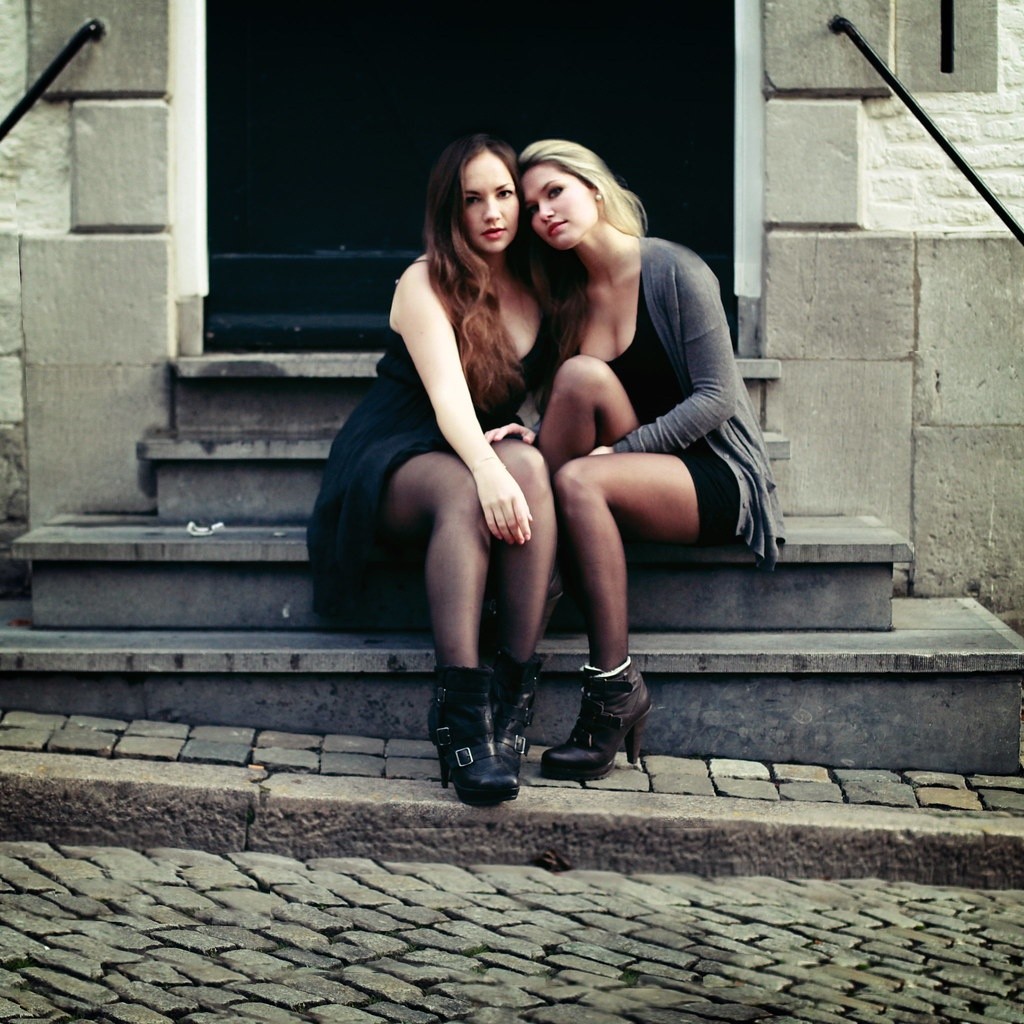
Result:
[428,647,543,805]
[541,655,652,781]
[478,563,563,646]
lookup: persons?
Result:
[303,135,560,808]
[478,140,786,778]
